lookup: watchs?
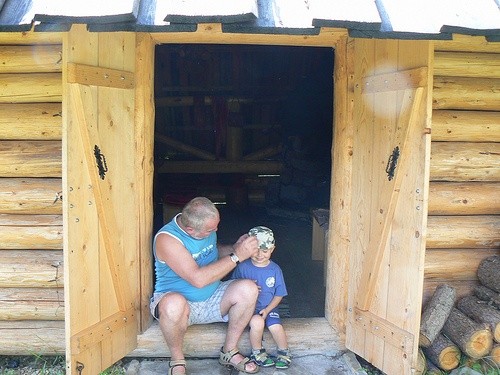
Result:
[229,253,240,265]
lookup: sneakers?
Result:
[275,349,292,369]
[249,348,275,367]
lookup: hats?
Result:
[247,226,275,250]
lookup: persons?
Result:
[230,226,293,369]
[150,197,259,375]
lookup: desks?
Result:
[161,159,287,175]
[310,208,329,286]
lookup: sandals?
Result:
[168,358,188,375]
[218,346,259,374]
